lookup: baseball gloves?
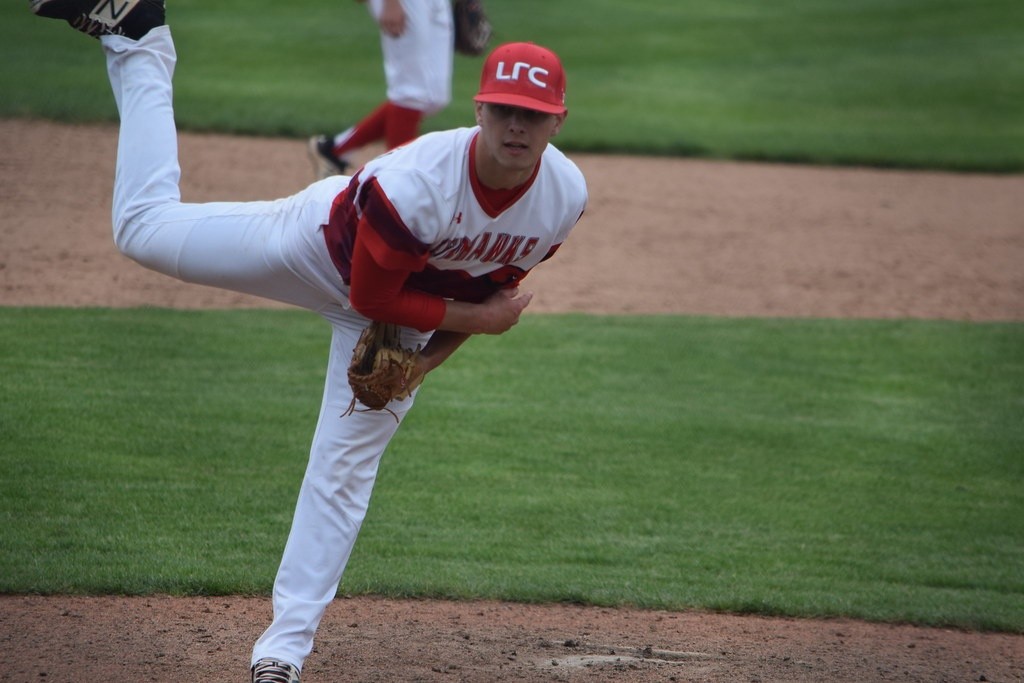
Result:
[345,321,426,411]
[453,2,495,57]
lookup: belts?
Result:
[322,224,343,275]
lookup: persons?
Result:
[30,0,587,683]
[306,0,490,182]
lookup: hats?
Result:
[472,41,566,114]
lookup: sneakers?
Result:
[31,0,166,40]
[252,657,301,683]
[308,134,348,183]
